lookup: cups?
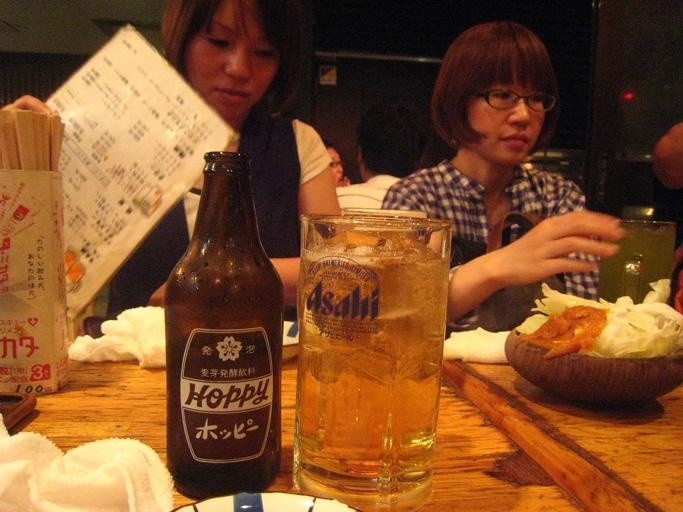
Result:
[595,220,676,306]
[292,212,452,509]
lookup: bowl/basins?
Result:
[504,328,683,409]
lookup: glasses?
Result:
[471,87,557,113]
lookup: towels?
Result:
[0,413,175,512]
[68,305,167,369]
[440,327,512,365]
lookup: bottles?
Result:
[165,152,284,500]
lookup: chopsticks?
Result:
[0,110,65,171]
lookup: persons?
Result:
[384,22,625,341]
[79,0,342,331]
[337,104,429,209]
[648,122,683,315]
[325,144,351,188]
